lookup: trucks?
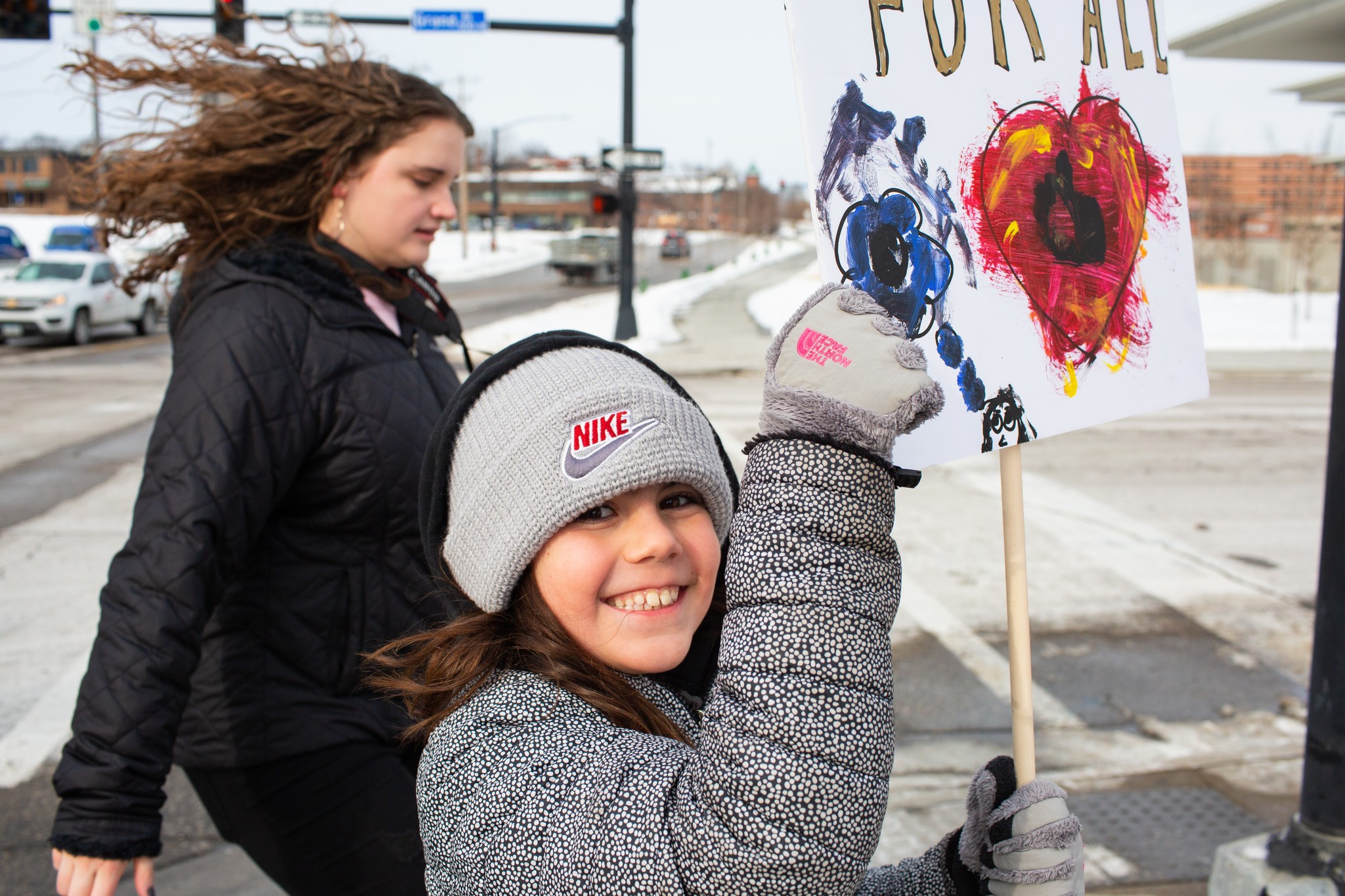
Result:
[548,235,634,283]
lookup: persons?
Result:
[361,282,1086,896]
[0,21,476,896]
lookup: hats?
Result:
[443,343,736,614]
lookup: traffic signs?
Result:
[601,143,664,175]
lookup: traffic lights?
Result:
[215,0,244,46]
[591,193,623,215]
[0,0,50,40]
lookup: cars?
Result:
[659,235,691,257]
[0,223,196,347]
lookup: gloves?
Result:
[760,281,946,464]
[944,755,1088,896]
